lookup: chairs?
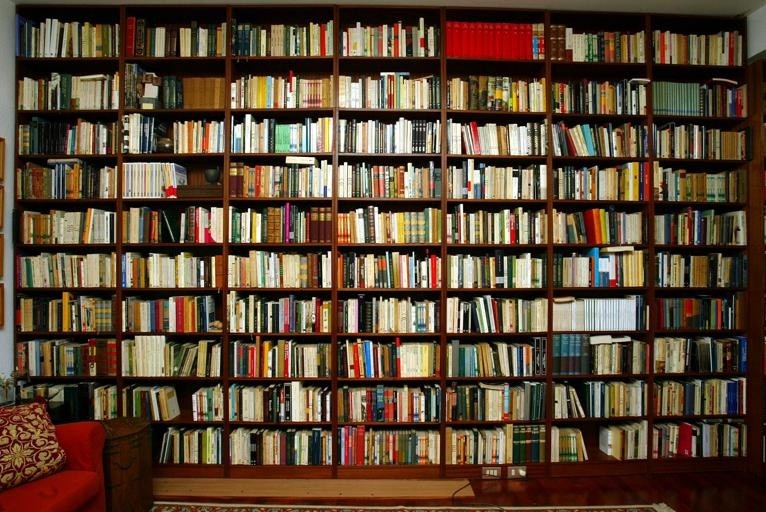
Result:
[0,422,107,512]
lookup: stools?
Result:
[98,417,154,512]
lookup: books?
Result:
[15,16,119,422]
[652,30,746,460]
[228,18,334,464]
[552,24,648,463]
[338,17,441,464]
[445,19,547,464]
[121,16,227,464]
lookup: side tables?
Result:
[0,400,69,424]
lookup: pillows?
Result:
[0,395,66,489]
